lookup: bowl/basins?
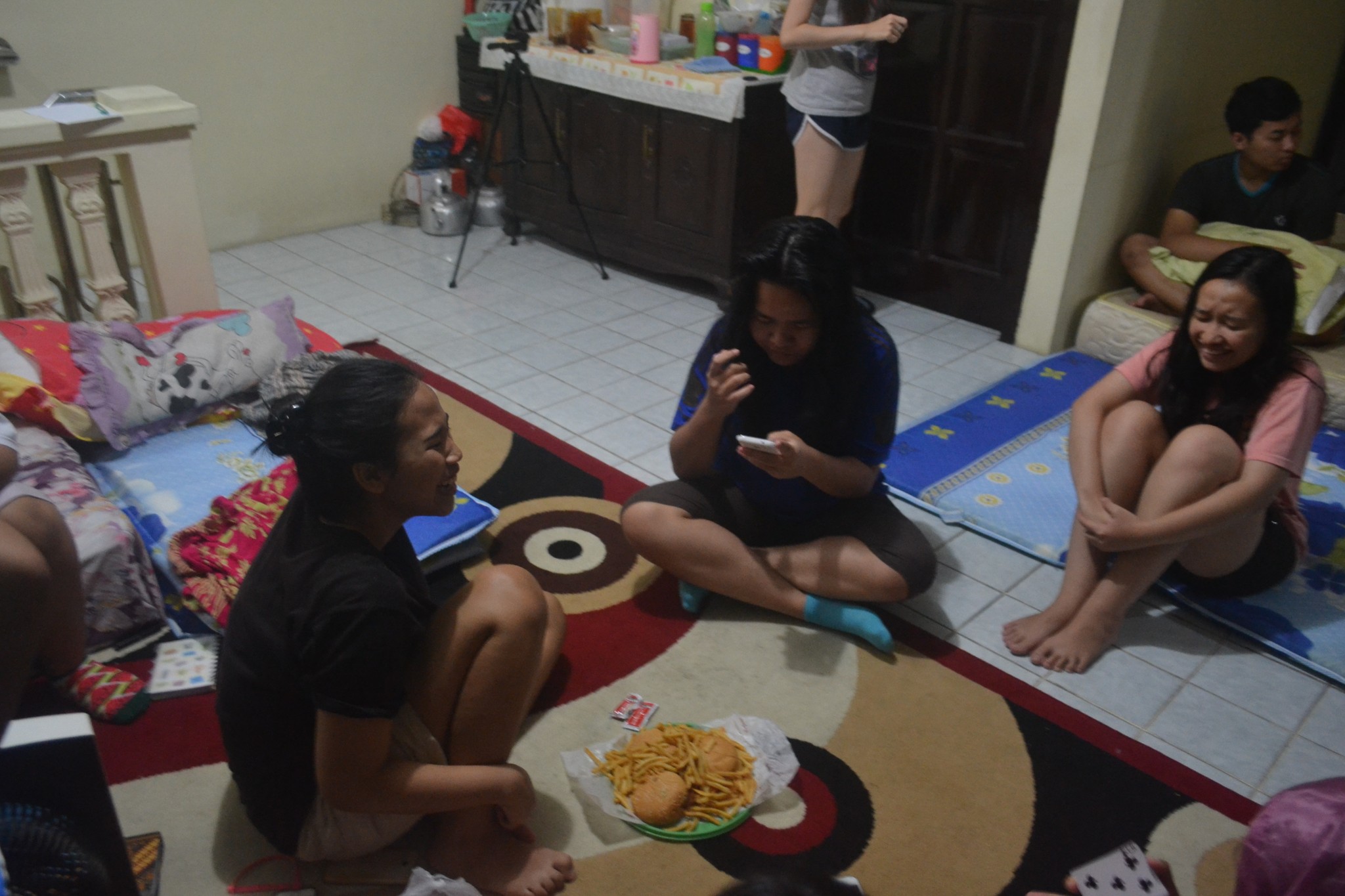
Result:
[461,12,511,42]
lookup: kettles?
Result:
[469,177,507,227]
[418,182,473,237]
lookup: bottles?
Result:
[751,12,770,34]
[628,0,660,63]
[695,3,715,60]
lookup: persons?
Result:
[0,437,150,732]
[777,1,913,217]
[1107,71,1343,335]
[995,242,1328,683]
[216,345,579,896]
[1024,776,1345,896]
[620,219,936,660]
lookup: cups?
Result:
[714,31,783,69]
[566,7,589,49]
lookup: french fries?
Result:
[583,726,757,834]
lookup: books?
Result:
[148,632,220,704]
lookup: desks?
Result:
[2,84,215,327]
[482,15,800,312]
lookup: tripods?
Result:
[450,56,610,291]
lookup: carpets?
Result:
[2,307,1344,895]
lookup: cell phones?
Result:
[736,435,778,457]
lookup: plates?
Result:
[611,721,757,842]
